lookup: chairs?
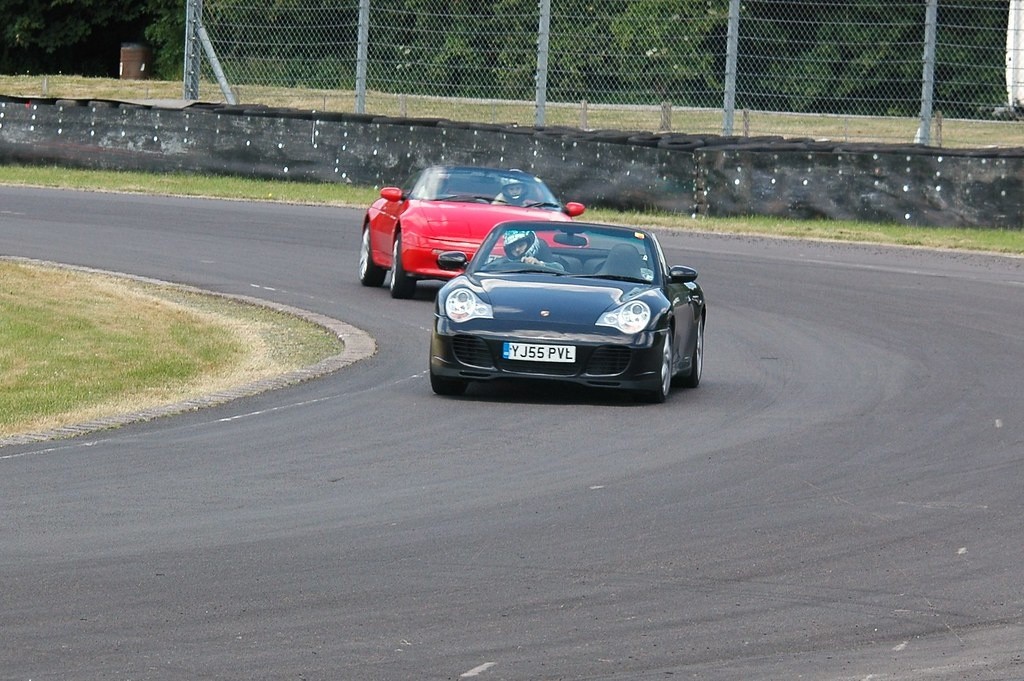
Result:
[591,242,645,281]
[535,238,573,276]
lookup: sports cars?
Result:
[427,219,707,404]
[357,162,589,299]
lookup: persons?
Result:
[503,229,570,273]
[499,168,544,208]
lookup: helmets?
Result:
[500,168,530,204]
[503,227,540,266]
